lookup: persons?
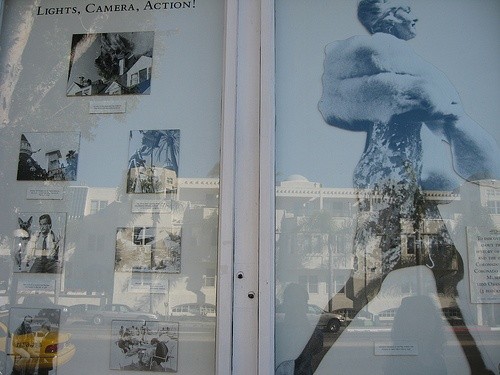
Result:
[272,0,500,375]
[117,323,174,368]
[22,214,59,272]
[12,315,56,375]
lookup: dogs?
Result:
[16,216,33,270]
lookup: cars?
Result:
[0,321,75,375]
[67,303,99,320]
[1,293,68,323]
[275,304,346,333]
[439,316,490,340]
[86,304,157,331]
